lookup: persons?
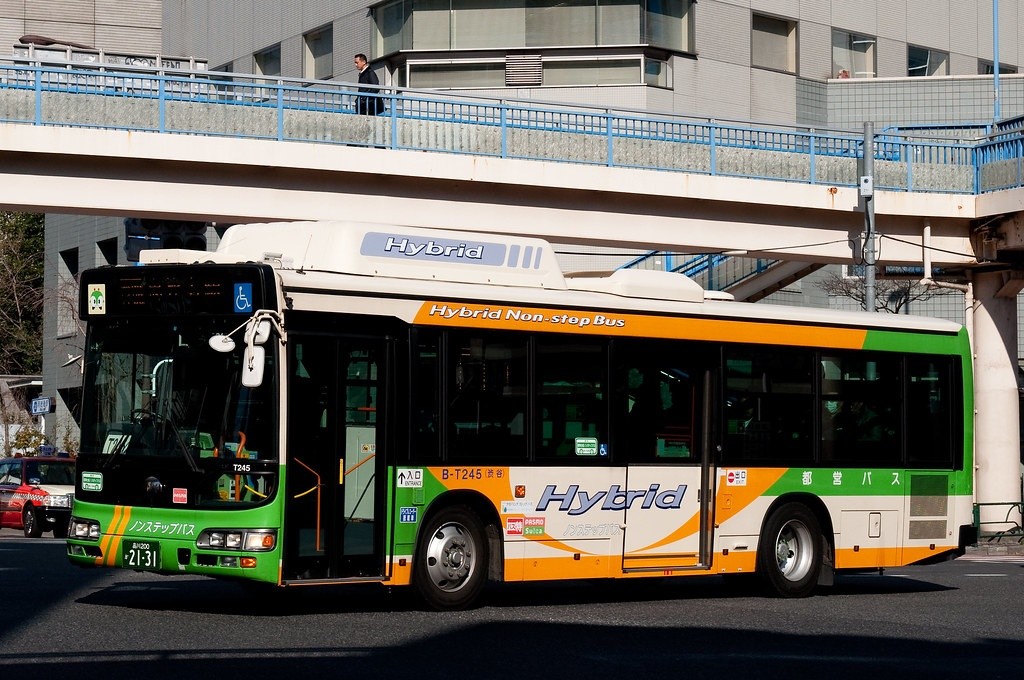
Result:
[347,54,387,149]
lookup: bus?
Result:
[56,220,979,616]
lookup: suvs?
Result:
[0,456,77,539]
